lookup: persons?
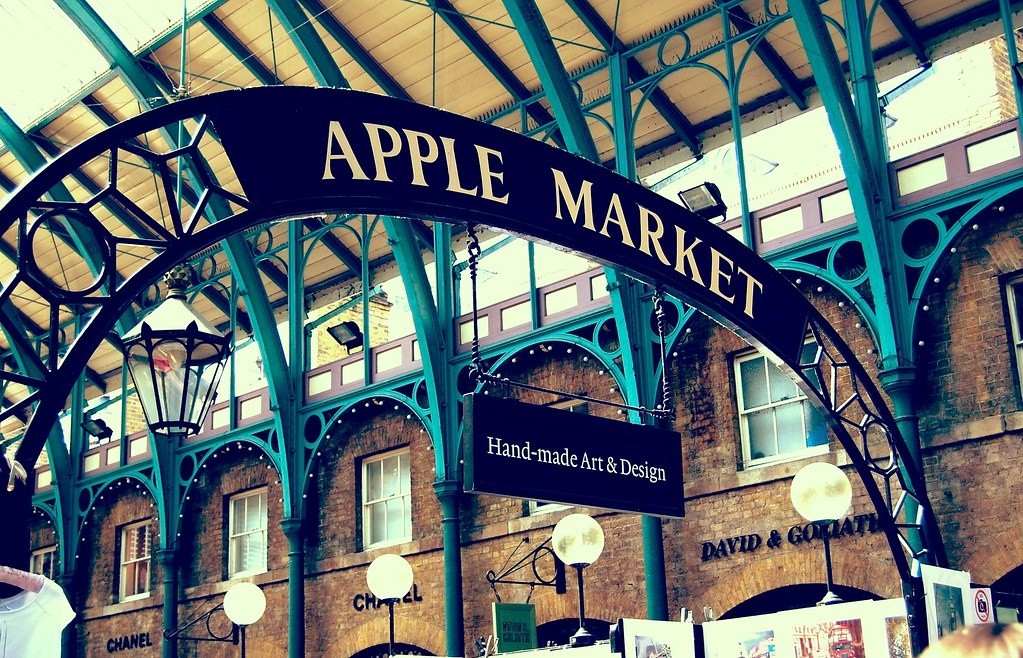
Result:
[917,623,1023,658]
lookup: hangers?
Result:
[0,565,45,595]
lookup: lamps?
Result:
[328,322,364,354]
[790,461,852,526]
[223,583,267,625]
[117,261,232,445]
[552,513,605,568]
[367,554,414,601]
[679,182,728,223]
[81,419,113,445]
